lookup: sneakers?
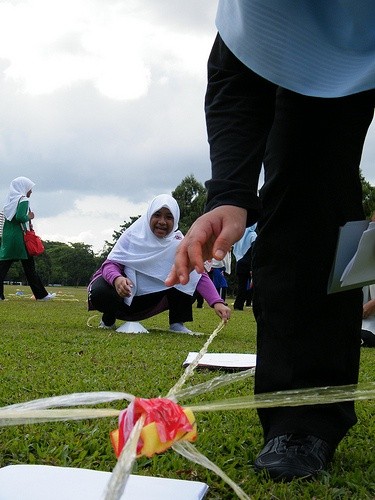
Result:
[254,436,296,467]
[269,435,334,481]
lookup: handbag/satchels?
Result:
[24,230,45,257]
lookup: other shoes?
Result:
[99,320,115,329]
[169,324,203,335]
[36,291,56,301]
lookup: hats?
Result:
[213,0,375,98]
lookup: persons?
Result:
[233,247,254,311]
[196,258,228,308]
[88,194,231,335]
[360,284,375,348]
[166,0,375,483]
[0,177,56,301]
